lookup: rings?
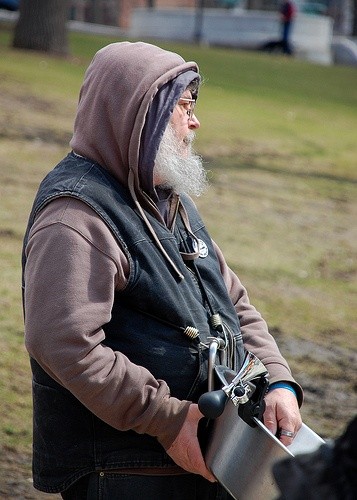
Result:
[279,428,294,437]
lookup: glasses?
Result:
[180,97,196,116]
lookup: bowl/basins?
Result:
[205,365,327,500]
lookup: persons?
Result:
[278,0,300,55]
[21,40,305,500]
